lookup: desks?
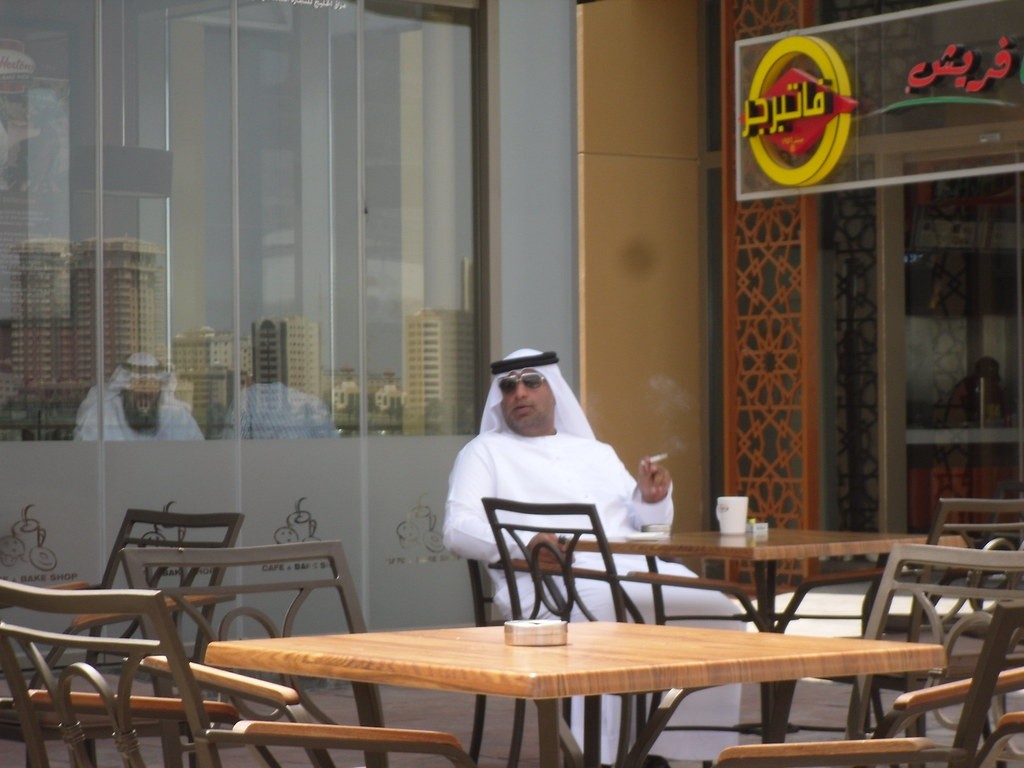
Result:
[204,616,947,767]
[565,529,971,760]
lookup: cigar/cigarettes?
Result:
[641,453,669,467]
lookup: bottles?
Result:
[749,518,757,532]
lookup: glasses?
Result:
[500,373,546,393]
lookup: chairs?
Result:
[1,495,1023,767]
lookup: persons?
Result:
[71,351,208,442]
[443,346,747,768]
[944,357,1013,428]
[222,370,341,439]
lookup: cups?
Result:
[754,523,768,531]
[716,497,749,534]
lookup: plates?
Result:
[624,532,670,540]
